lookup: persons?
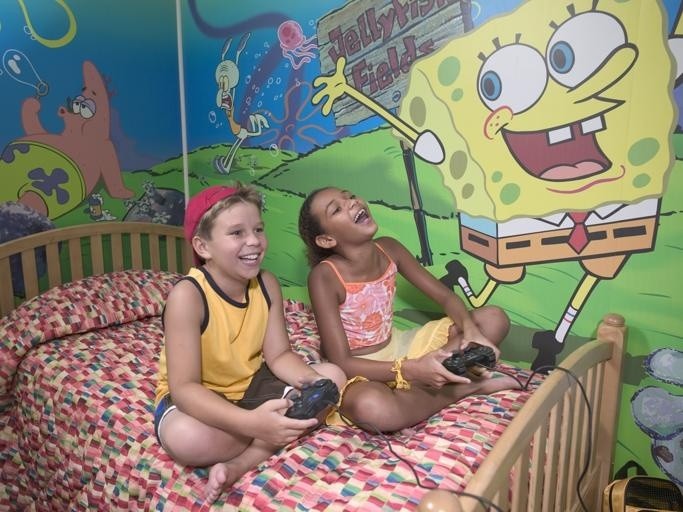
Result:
[298,185,535,434]
[153,182,347,505]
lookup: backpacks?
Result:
[601,460,683,512]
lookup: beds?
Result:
[0,222,630,511]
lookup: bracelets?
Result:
[391,354,411,391]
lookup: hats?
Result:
[182,183,240,271]
